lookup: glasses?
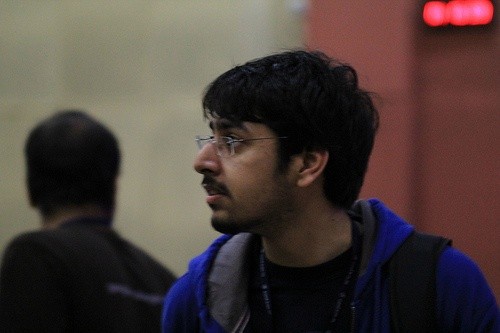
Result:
[195,135,287,157]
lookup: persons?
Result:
[160,51,500,333]
[0,108,180,332]
